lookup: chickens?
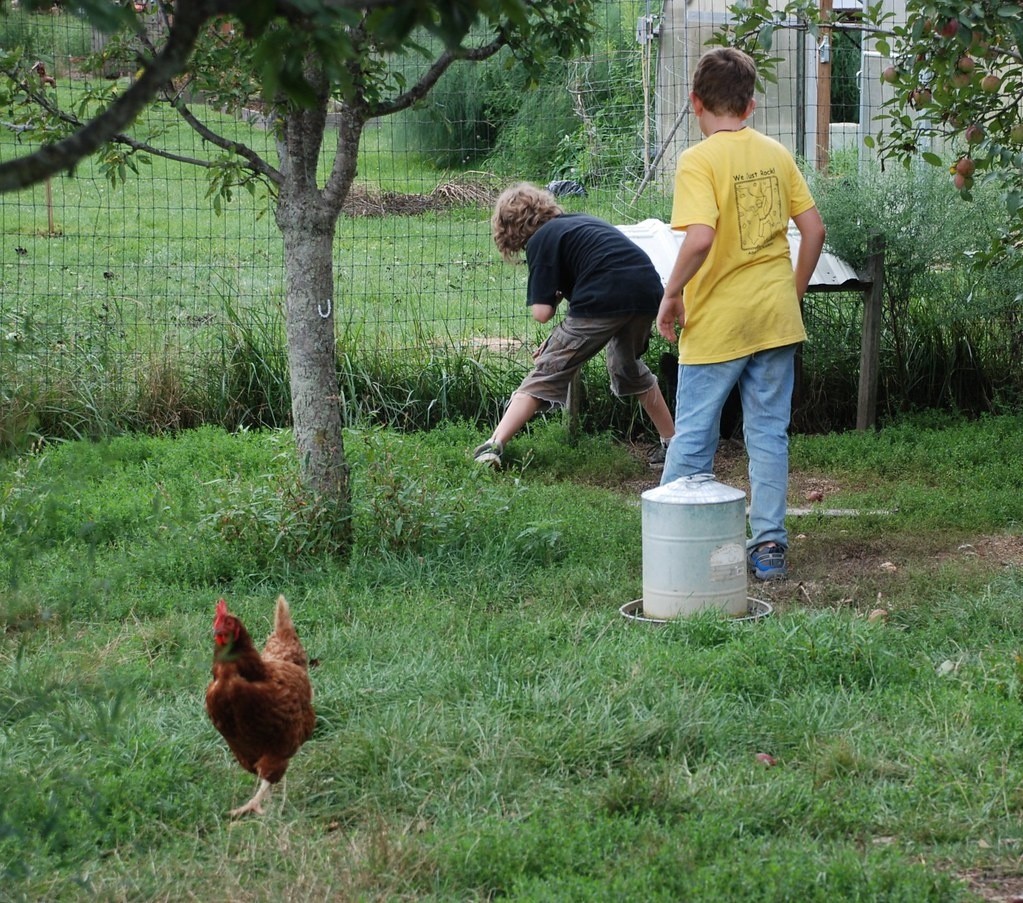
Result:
[206,594,315,821]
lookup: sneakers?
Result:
[750,545,788,581]
[473,443,503,471]
[650,447,669,469]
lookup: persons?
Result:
[472,47,826,581]
